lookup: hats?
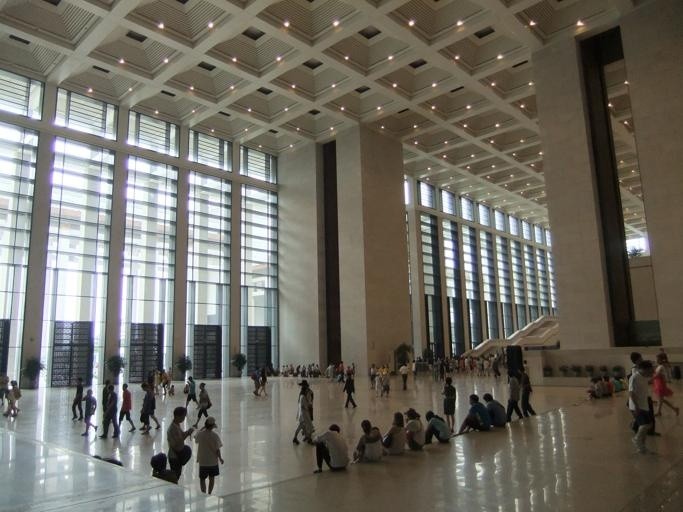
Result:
[206,416,218,428]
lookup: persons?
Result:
[423,410,452,444]
[382,413,407,455]
[518,366,536,418]
[0,369,22,418]
[586,348,680,455]
[402,408,426,450]
[441,376,456,432]
[370,349,537,398]
[483,393,508,427]
[459,395,491,432]
[250,358,357,444]
[353,420,384,464]
[506,368,523,422]
[73,365,224,495]
[311,424,350,473]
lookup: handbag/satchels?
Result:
[178,445,191,466]
